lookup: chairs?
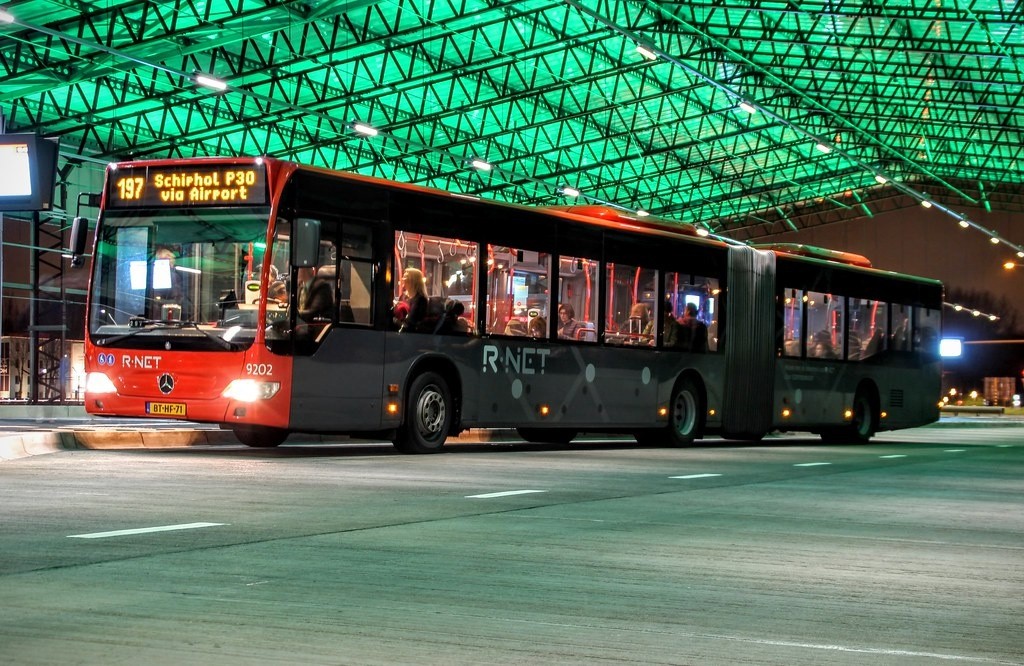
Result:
[316,264,343,323]
[419,295,465,335]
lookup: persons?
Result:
[393,268,429,333]
[298,267,333,311]
[620,301,708,349]
[454,299,469,332]
[255,265,288,303]
[895,319,909,340]
[557,304,580,340]
[813,344,836,358]
[152,249,186,322]
[529,317,546,338]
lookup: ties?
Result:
[299,283,306,310]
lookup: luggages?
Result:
[628,316,643,347]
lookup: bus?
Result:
[69,156,943,455]
